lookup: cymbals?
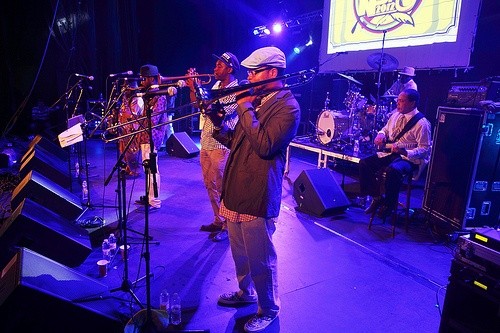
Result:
[337,72,363,85]
[367,53,399,72]
[380,95,398,98]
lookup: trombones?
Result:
[101,65,317,143]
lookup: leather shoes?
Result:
[363,195,384,215]
[378,201,398,217]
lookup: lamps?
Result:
[293,33,314,54]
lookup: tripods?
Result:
[44,78,210,333]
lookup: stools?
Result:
[369,166,418,237]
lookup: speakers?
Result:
[18,149,71,189]
[11,170,83,220]
[0,247,118,333]
[0,199,91,267]
[439,259,500,333]
[422,106,500,229]
[293,167,351,217]
[166,131,200,158]
[20,144,62,164]
[28,135,68,160]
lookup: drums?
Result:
[316,108,367,146]
[366,105,388,115]
[344,90,368,110]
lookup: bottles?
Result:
[160,289,170,317]
[171,292,181,325]
[325,92,330,110]
[102,239,110,263]
[75,163,79,177]
[82,180,88,200]
[108,234,117,257]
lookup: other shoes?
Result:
[212,229,229,241]
[136,205,162,212]
[134,198,149,205]
[201,222,222,232]
[217,289,258,304]
[243,311,281,333]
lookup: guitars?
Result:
[375,139,418,158]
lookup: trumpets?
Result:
[158,71,216,88]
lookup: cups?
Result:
[96,260,107,276]
[120,245,130,260]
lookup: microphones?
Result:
[75,73,94,80]
[152,80,186,89]
[134,86,177,96]
[109,71,133,77]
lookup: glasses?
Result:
[246,66,275,74]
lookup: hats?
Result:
[212,51,240,70]
[122,80,139,90]
[240,47,287,69]
[138,65,159,77]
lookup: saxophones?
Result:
[104,86,145,134]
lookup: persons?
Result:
[185,52,240,241]
[358,89,432,220]
[204,47,300,331]
[117,64,175,212]
[384,66,417,102]
